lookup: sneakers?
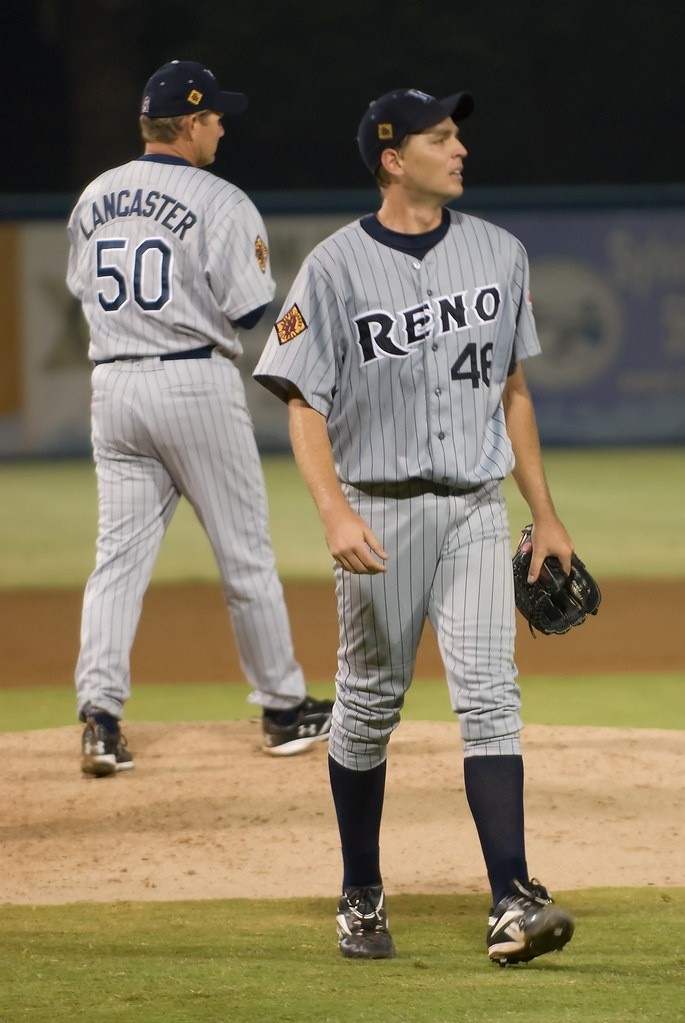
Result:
[261,697,337,756]
[486,878,574,968]
[79,712,134,775]
[334,886,395,959]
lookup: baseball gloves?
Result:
[511,523,602,637]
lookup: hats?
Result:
[141,60,247,118]
[357,88,470,176]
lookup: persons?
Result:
[66,61,335,776]
[252,89,602,968]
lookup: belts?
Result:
[94,344,216,367]
